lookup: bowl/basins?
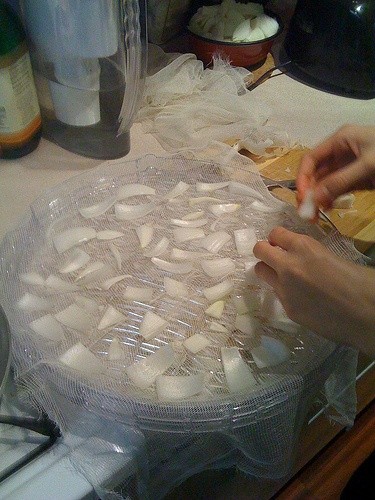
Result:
[183,7,286,68]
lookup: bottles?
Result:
[18,0,150,161]
[0,0,45,160]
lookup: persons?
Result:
[253,124,375,362]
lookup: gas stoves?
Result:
[0,381,148,500]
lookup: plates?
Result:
[1,153,367,435]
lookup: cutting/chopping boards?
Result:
[224,136,375,262]
